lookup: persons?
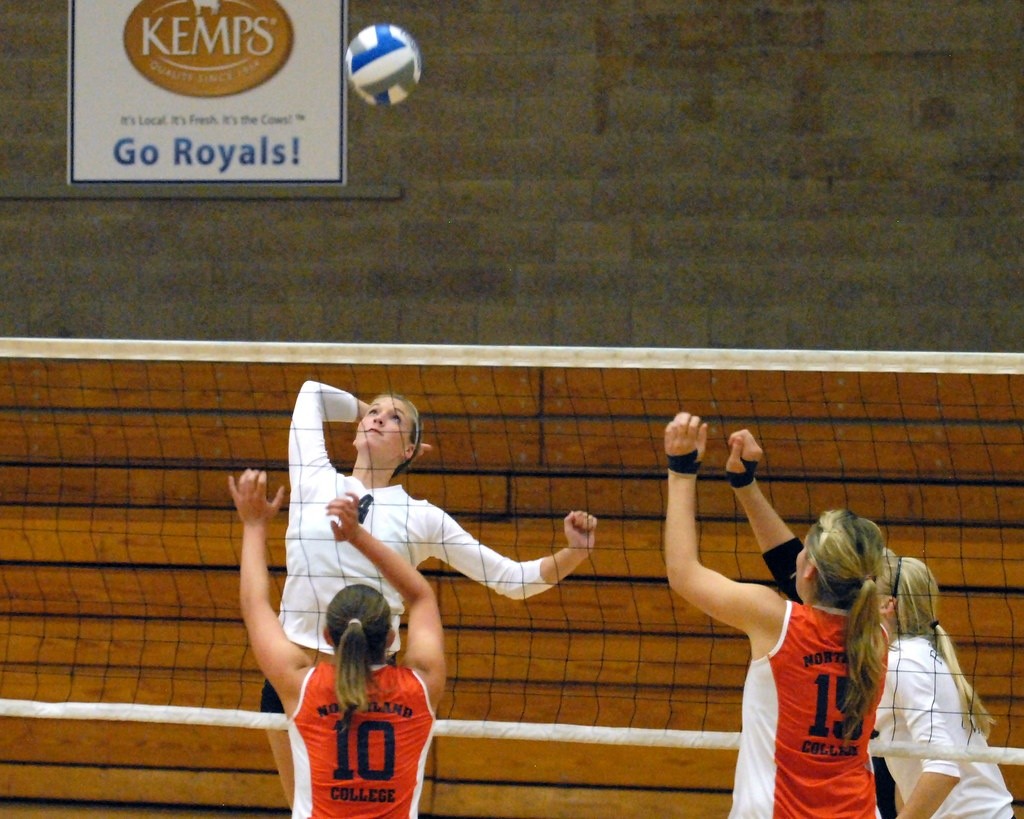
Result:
[260,380,599,811]
[662,411,893,819]
[225,467,449,819]
[865,547,1020,819]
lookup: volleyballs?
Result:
[344,20,423,108]
